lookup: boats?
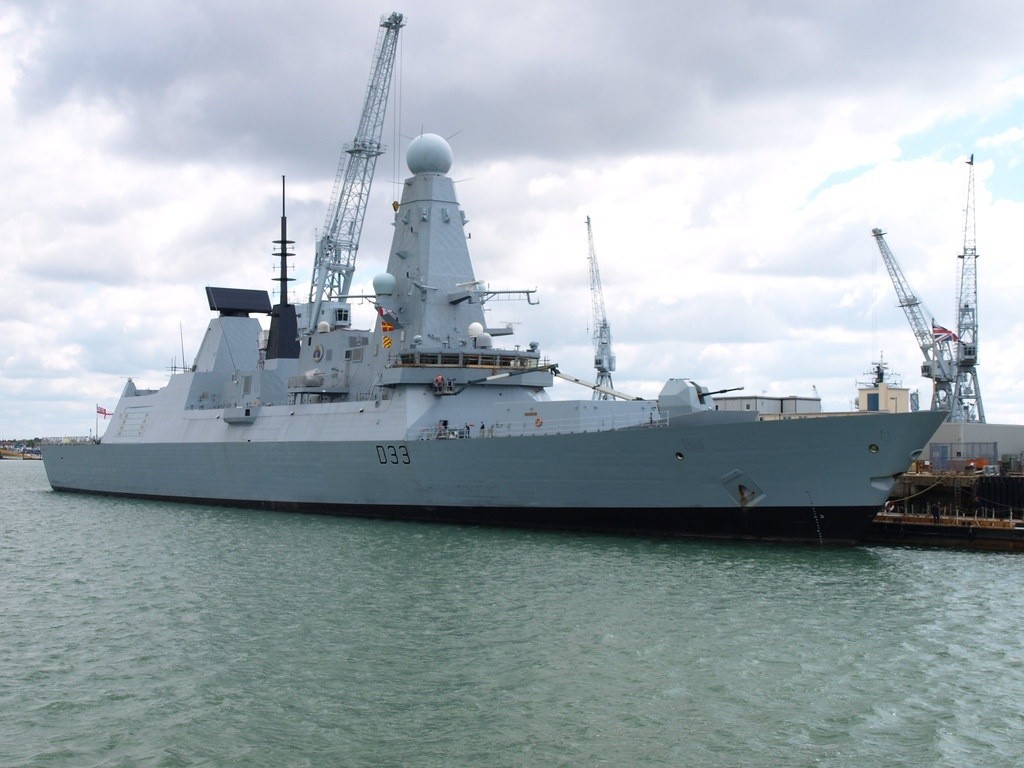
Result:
[36,126,954,532]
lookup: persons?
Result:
[932,505,939,524]
[464,423,471,438]
[436,374,457,392]
[873,366,884,388]
[480,421,494,438]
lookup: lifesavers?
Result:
[534,416,543,428]
[885,500,895,513]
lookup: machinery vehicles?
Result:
[870,152,989,423]
[290,11,410,336]
[583,216,618,400]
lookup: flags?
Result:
[932,322,966,347]
[381,321,394,332]
[382,336,392,349]
[379,307,393,315]
[97,407,106,416]
